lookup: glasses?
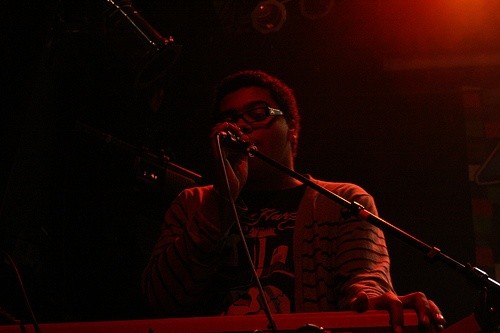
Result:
[209,103,286,129]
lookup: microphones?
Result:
[217,128,235,147]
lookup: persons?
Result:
[141,70,447,333]
